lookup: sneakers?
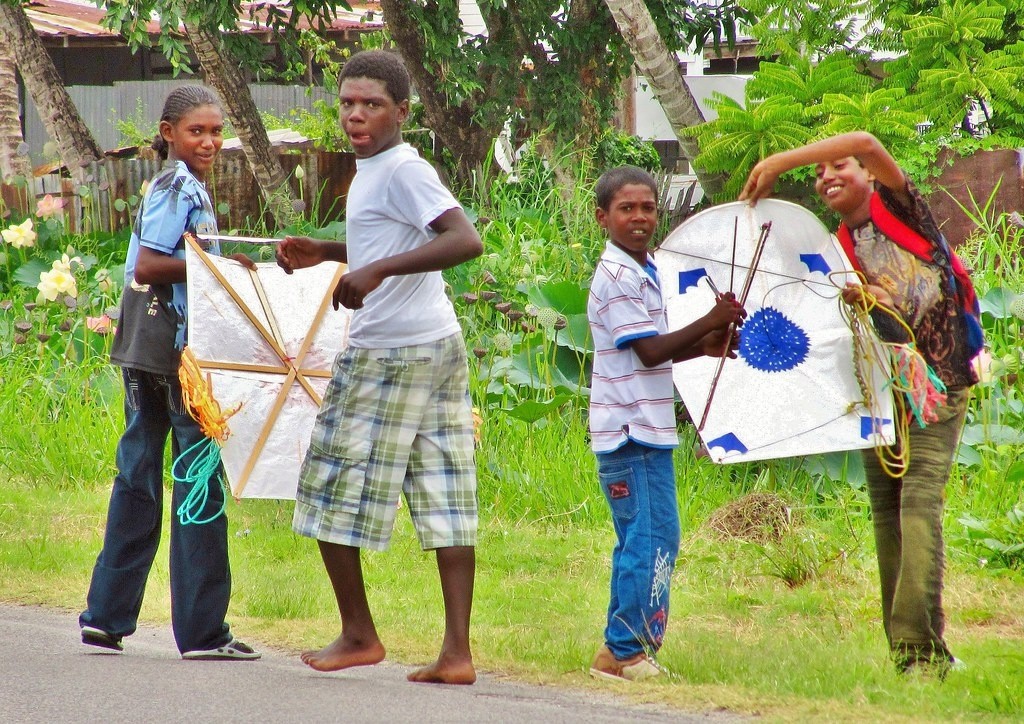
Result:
[590,641,676,687]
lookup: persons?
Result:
[77,85,260,660]
[736,129,983,685]
[585,168,746,687]
[274,49,484,686]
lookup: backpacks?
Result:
[838,190,985,363]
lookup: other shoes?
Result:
[904,663,936,688]
[949,658,963,677]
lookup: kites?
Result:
[182,232,355,505]
[646,198,896,463]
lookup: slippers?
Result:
[82,627,125,650]
[182,637,262,660]
